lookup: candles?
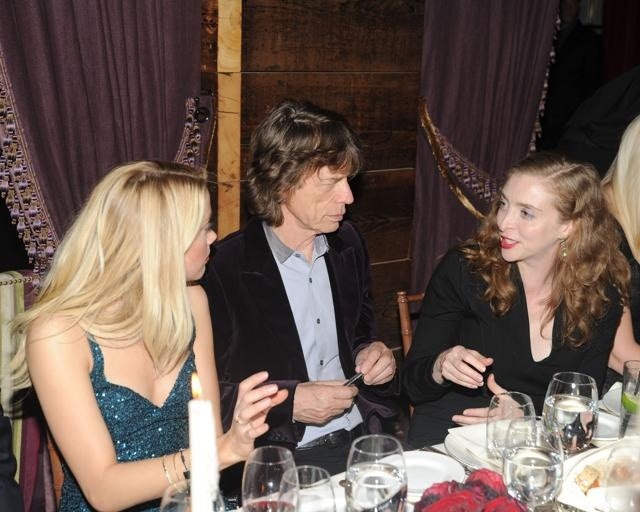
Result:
[187,371,217,512]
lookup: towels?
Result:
[447,422,503,469]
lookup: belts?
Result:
[301,422,365,450]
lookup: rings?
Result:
[234,412,245,426]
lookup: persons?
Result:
[394,143,640,450]
[9,157,291,512]
[597,116,640,269]
[0,398,26,511]
[186,100,402,506]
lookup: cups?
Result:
[485,391,537,468]
[502,415,565,507]
[241,433,337,512]
[618,359,640,440]
[159,478,226,511]
[345,433,408,511]
[541,371,599,454]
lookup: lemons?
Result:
[622,392,639,415]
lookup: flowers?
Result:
[414,469,528,512]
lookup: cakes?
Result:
[576,456,640,496]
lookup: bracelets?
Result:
[177,446,191,481]
[171,451,188,495]
[163,452,184,495]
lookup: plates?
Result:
[592,411,620,441]
[443,433,481,470]
[377,450,467,503]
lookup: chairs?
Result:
[397,290,426,415]
[45,426,64,510]
